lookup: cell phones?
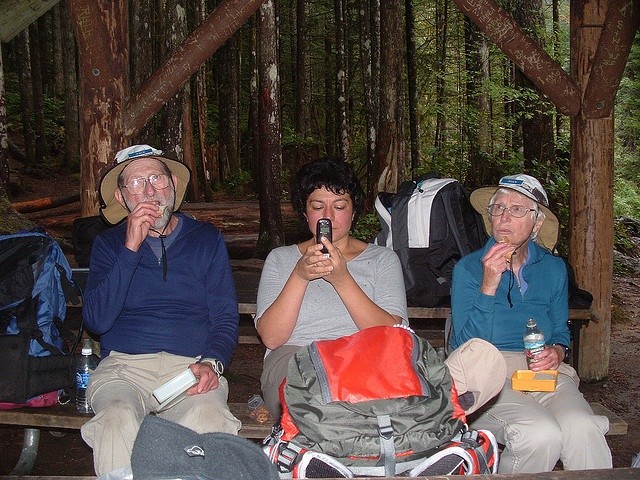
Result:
[316,217,332,253]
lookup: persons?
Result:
[254,158,410,424]
[81,144,245,480]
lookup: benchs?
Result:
[0,291,630,479]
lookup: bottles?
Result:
[73,338,98,420]
[523,320,546,374]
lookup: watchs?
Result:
[199,358,224,378]
[447,174,613,474]
[556,342,571,364]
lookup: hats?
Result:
[469,174,559,252]
[98,144,191,227]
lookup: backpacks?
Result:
[373,172,488,308]
[0,226,74,403]
[264,325,500,453]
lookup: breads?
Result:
[497,236,516,263]
[153,204,167,218]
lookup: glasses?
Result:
[486,204,536,218]
[122,173,173,195]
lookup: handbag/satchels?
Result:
[263,422,501,479]
[131,414,280,478]
